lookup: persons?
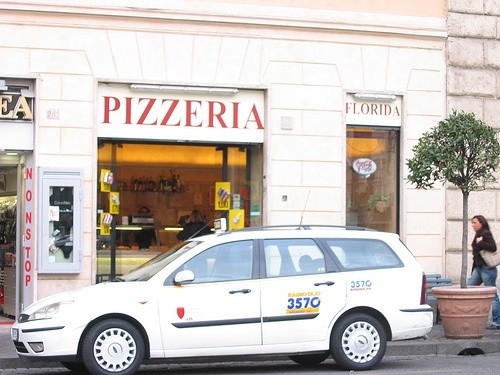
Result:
[467,215,500,330]
[176,209,210,275]
[134,206,163,250]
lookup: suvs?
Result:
[9,224,435,375]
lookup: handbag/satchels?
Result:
[479,245,500,269]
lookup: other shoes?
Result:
[485,324,500,330]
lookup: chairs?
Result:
[297,256,313,275]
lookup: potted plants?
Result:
[405,109,500,339]
[368,195,390,214]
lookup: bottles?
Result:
[123,182,127,190]
[132,174,182,192]
[118,180,121,189]
